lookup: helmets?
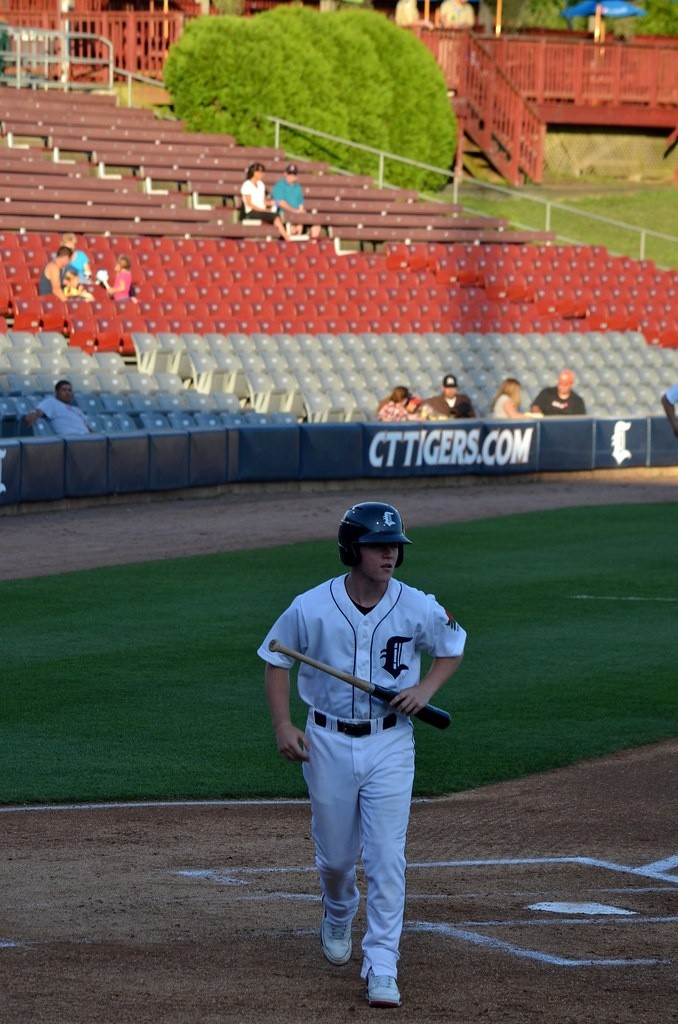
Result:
[339,502,412,568]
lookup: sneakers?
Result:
[321,916,352,966]
[366,967,402,1006]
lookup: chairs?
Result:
[0,230,678,435]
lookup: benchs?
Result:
[0,87,555,251]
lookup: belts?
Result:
[315,710,397,738]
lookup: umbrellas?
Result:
[559,0,645,19]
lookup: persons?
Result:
[530,370,586,417]
[257,501,467,1007]
[376,375,476,422]
[395,0,475,31]
[25,380,94,435]
[240,162,307,244]
[661,381,678,439]
[491,378,530,418]
[38,233,132,305]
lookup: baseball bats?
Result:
[267,640,453,730]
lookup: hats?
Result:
[558,370,574,385]
[287,163,297,172]
[443,375,456,387]
